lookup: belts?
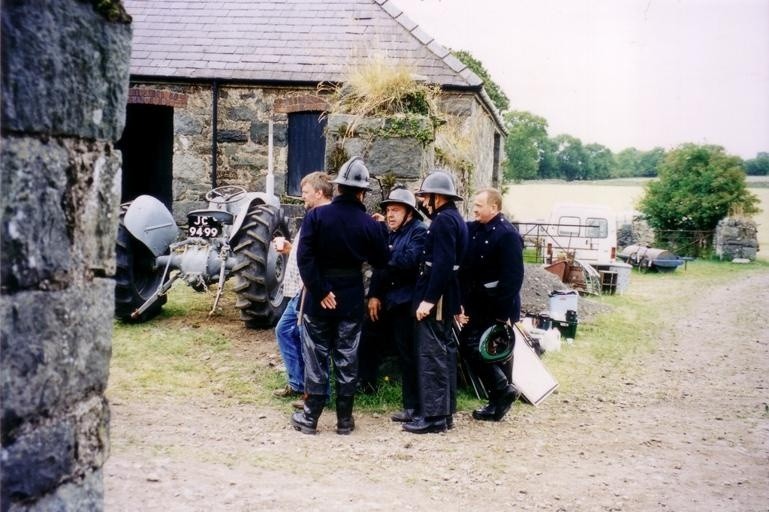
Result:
[423,260,462,271]
[481,277,502,291]
[323,265,363,280]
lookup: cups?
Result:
[275,237,287,252]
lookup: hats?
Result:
[328,155,374,191]
[416,170,463,200]
[379,188,425,222]
[477,323,517,363]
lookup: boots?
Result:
[291,392,326,435]
[335,394,355,435]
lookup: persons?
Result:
[403,170,469,432]
[291,154,389,434]
[464,187,525,421]
[366,181,430,420]
[271,170,334,408]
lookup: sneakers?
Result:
[292,399,305,410]
[273,387,303,397]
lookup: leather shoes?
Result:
[493,380,519,421]
[390,413,411,421]
[414,415,453,429]
[474,404,494,420]
[402,418,448,434]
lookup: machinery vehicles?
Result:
[113,185,291,334]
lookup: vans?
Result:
[538,203,620,296]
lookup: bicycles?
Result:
[626,241,655,276]
